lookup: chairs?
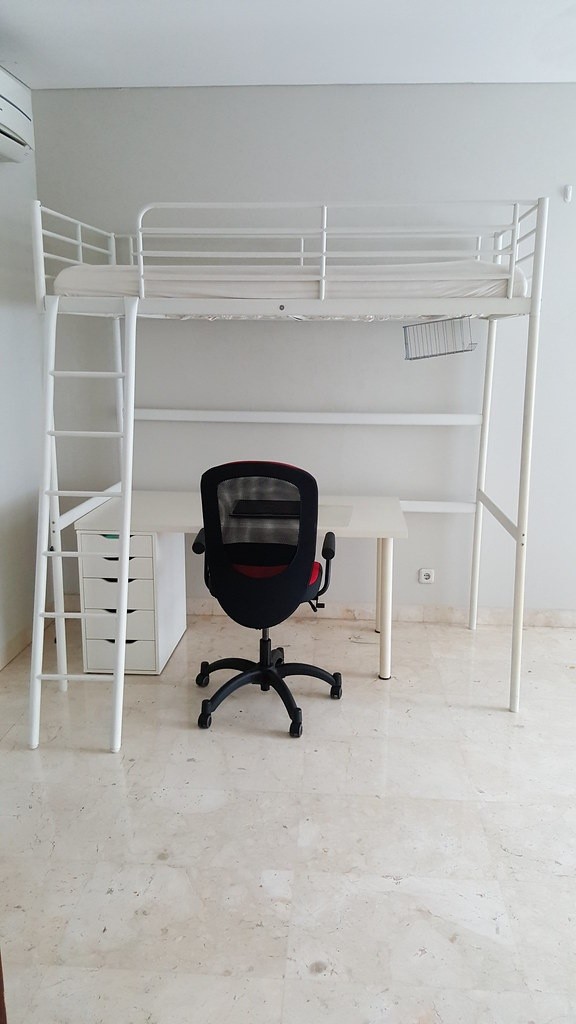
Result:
[190,459,346,737]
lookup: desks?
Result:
[73,485,403,685]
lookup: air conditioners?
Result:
[0,92,36,163]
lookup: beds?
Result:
[29,194,550,722]
